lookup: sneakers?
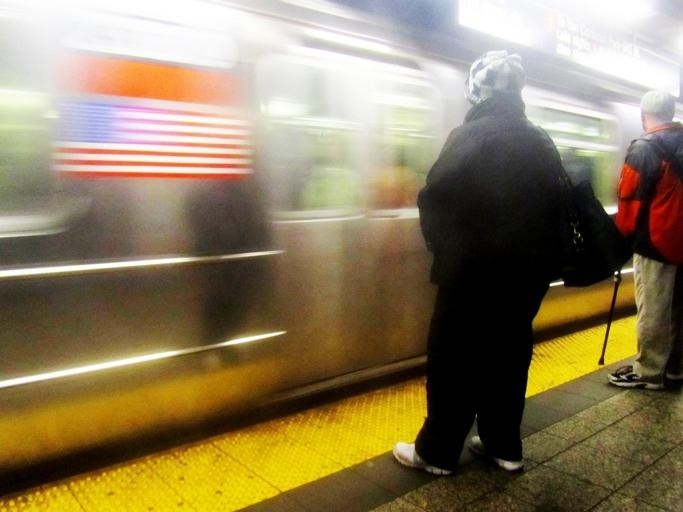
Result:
[393,442,452,476]
[607,366,683,390]
[468,436,524,471]
[666,369,683,379]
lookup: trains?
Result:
[0,0,683,476]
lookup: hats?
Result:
[466,50,526,105]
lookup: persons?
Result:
[606,90,683,391]
[393,52,571,476]
[175,125,415,352]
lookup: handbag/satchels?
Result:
[550,178,634,286]
